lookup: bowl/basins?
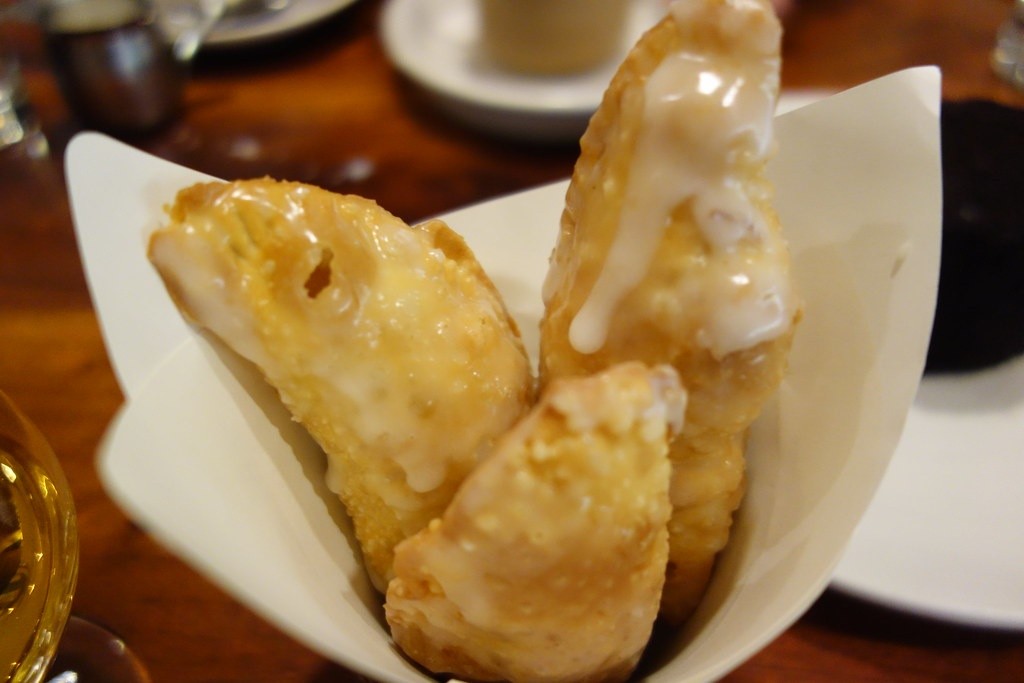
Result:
[66,62,944,683]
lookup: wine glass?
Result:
[0,392,151,682]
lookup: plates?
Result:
[771,92,1023,637]
[154,1,354,49]
[381,1,672,134]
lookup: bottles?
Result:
[38,0,183,140]
